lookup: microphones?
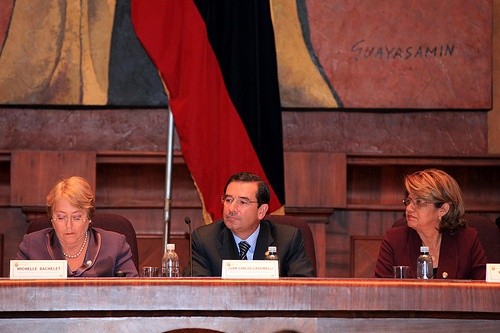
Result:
[185,216,193,277]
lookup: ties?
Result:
[238,240,250,260]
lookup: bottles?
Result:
[265,246,280,275]
[162,244,179,277]
[417,246,433,280]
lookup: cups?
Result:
[393,266,409,279]
[143,267,158,277]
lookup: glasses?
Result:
[403,197,444,206]
[50,214,91,226]
[221,194,258,206]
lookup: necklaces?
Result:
[64,232,87,258]
[422,236,442,262]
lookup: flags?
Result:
[130,0,285,225]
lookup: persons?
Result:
[15,176,140,277]
[182,172,315,277]
[374,169,488,280]
